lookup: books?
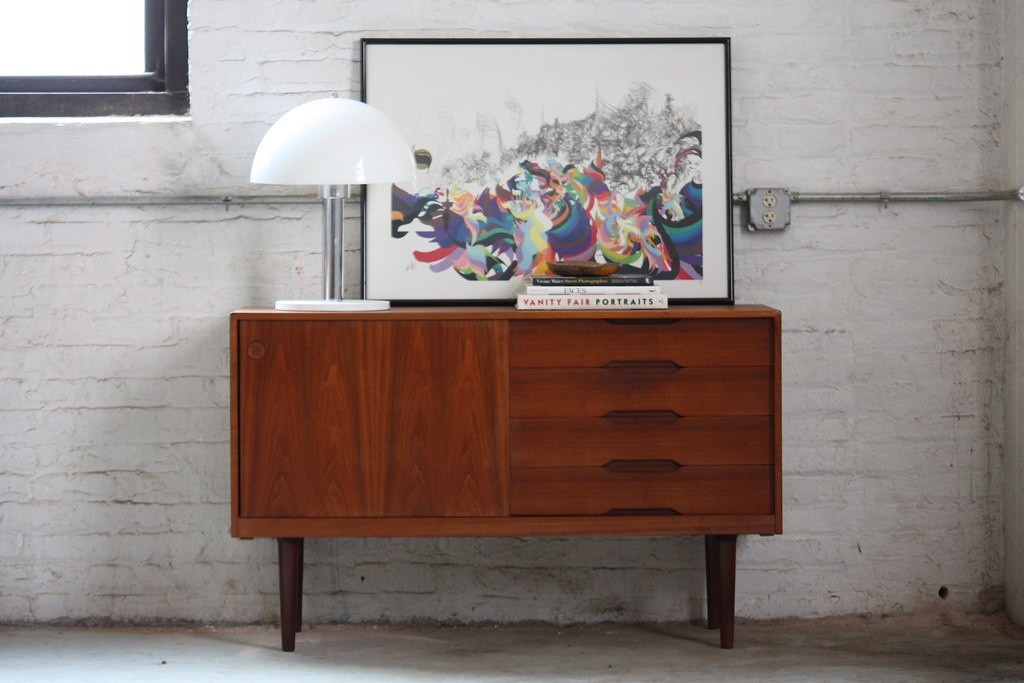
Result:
[514,273,668,310]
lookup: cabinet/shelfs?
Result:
[227,305,791,653]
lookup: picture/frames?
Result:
[360,36,736,307]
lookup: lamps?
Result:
[248,95,415,313]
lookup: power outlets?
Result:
[746,186,792,231]
